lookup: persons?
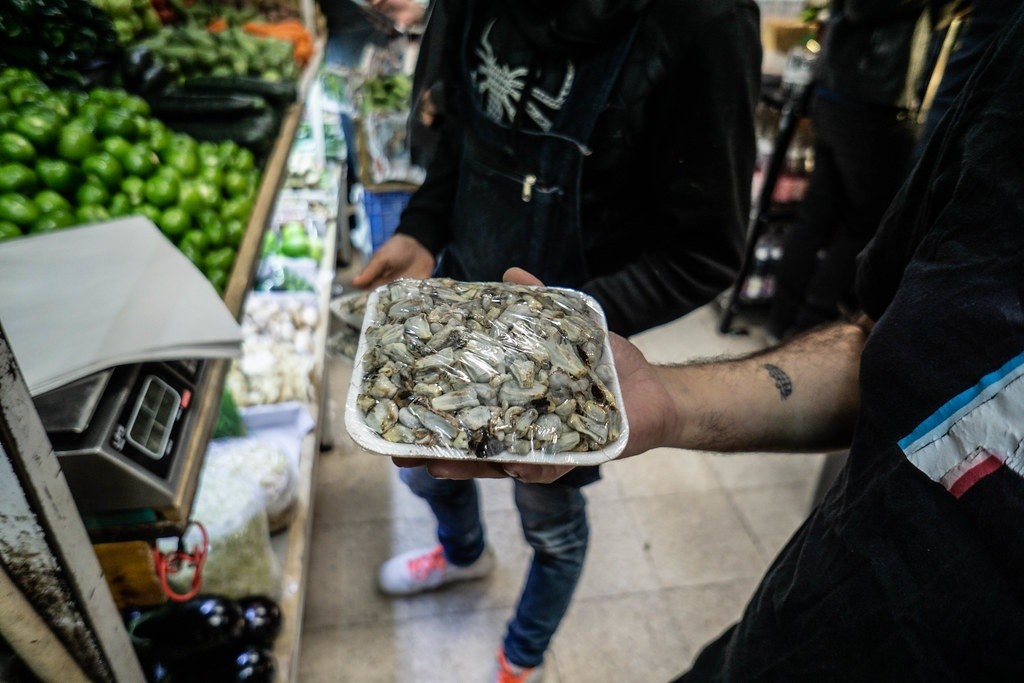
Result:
[318,0,426,269]
[347,0,763,683]
[391,0,1024,683]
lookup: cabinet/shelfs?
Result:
[0,15,359,683]
[716,68,815,333]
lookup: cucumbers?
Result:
[361,72,413,111]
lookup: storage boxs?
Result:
[761,15,820,54]
[364,192,413,251]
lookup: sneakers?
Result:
[377,543,495,594]
[494,647,545,683]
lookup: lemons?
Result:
[263,222,323,260]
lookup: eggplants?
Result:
[116,594,282,683]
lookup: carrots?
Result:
[209,18,314,60]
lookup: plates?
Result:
[342,277,630,467]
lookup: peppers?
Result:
[0,68,259,297]
[91,0,301,89]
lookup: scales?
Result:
[27,358,208,510]
